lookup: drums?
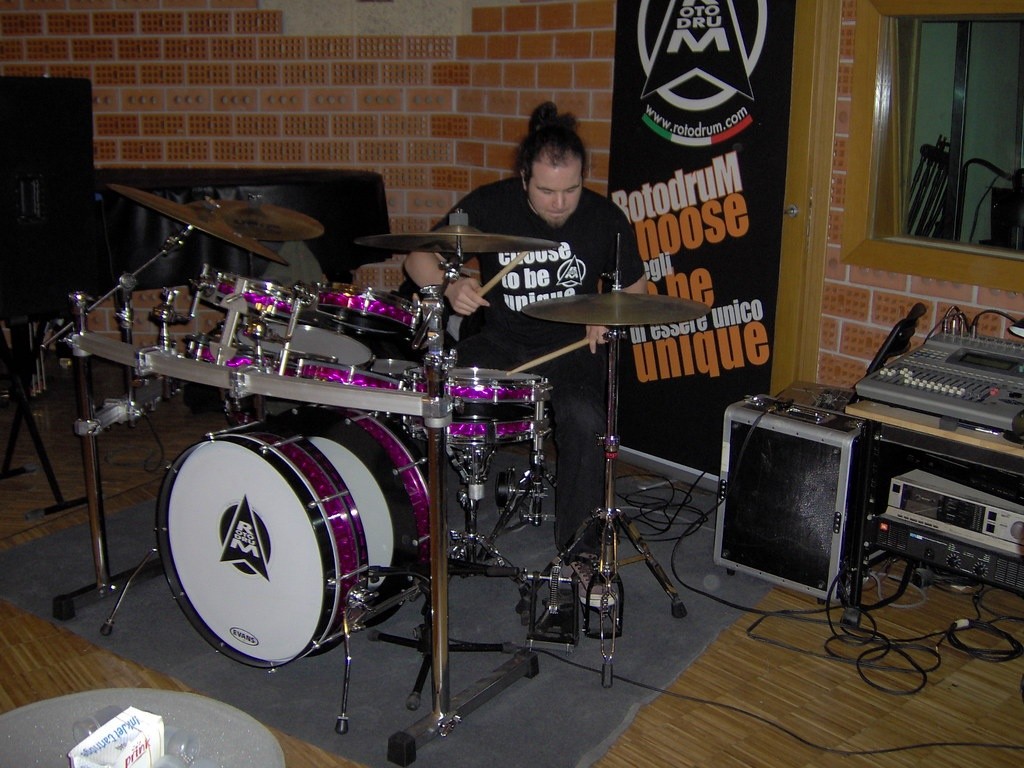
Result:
[296,356,402,391]
[156,406,431,666]
[212,272,299,322]
[236,322,377,372]
[317,281,421,335]
[368,354,418,375]
[405,365,548,450]
[182,333,295,378]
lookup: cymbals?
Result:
[522,291,711,325]
[351,225,562,255]
[185,196,325,243]
[107,183,291,268]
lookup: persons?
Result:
[401,101,650,608]
